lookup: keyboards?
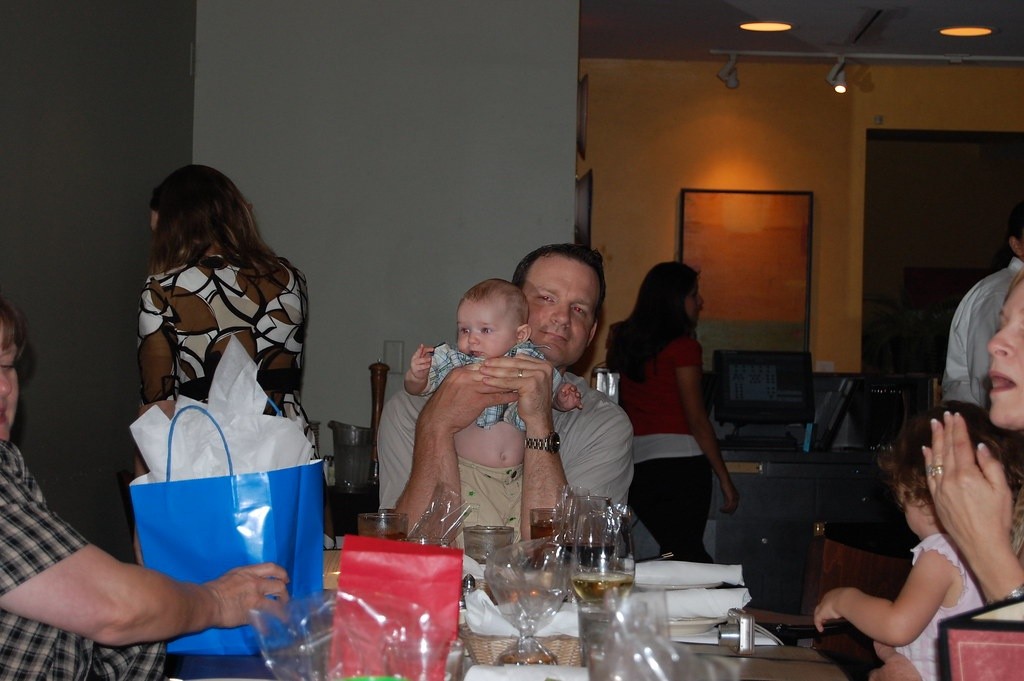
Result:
[719,437,797,451]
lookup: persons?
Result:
[607,261,738,563]
[376,242,633,539]
[814,203,1024,681]
[132,165,306,569]
[405,278,583,545]
[1,297,290,680]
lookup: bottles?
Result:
[322,455,335,486]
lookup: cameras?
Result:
[718,608,755,656]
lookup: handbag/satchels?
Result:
[128,395,323,654]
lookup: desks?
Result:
[158,547,850,681]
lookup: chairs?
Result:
[800,518,913,676]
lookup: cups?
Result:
[356,513,409,543]
[462,525,515,565]
[564,496,635,666]
[529,508,558,560]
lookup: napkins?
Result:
[464,586,582,639]
[632,586,751,620]
[632,560,745,588]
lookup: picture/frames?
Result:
[676,189,814,374]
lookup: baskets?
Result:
[459,623,582,668]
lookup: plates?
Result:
[636,582,724,590]
[669,618,728,638]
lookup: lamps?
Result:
[826,58,847,93]
[716,56,739,89]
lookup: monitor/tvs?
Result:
[711,349,815,426]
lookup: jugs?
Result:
[328,421,373,495]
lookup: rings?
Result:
[927,465,943,475]
[518,369,523,377]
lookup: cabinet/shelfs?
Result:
[629,449,913,629]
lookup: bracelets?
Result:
[1007,585,1024,600]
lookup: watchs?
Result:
[524,433,560,454]
